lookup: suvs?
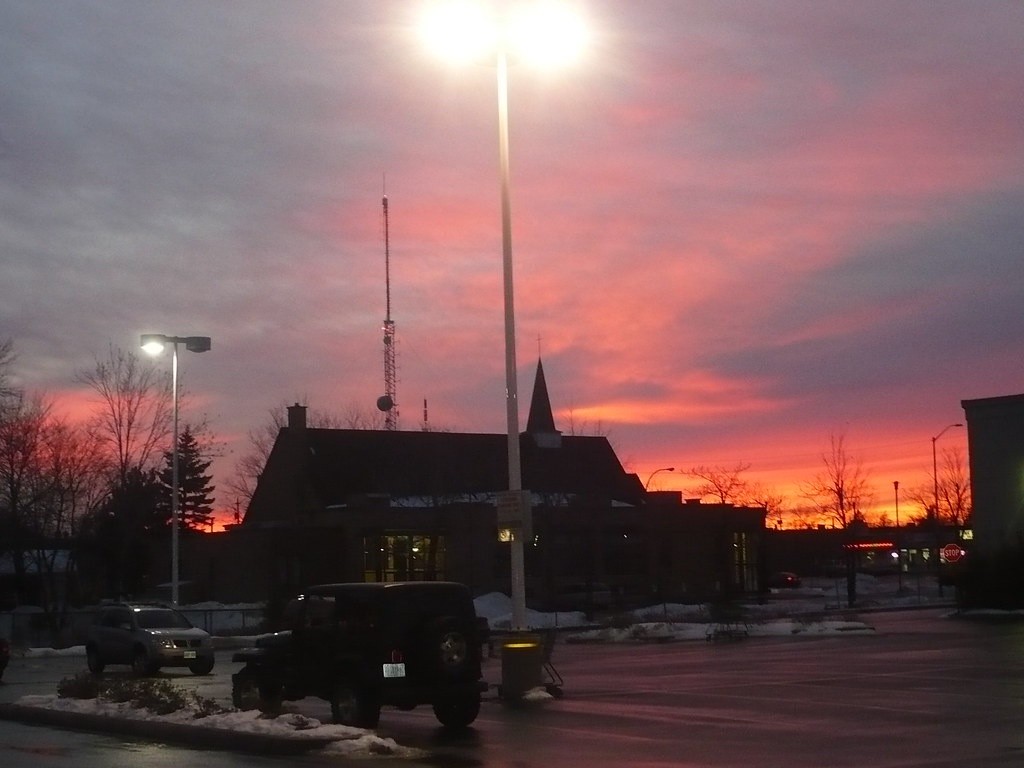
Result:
[85,603,216,677]
[230,580,492,728]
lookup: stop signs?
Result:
[943,544,962,561]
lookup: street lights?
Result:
[892,481,903,591]
[931,423,964,596]
[139,333,210,610]
[646,467,674,491]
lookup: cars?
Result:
[774,571,801,587]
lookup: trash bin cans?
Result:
[497,632,545,702]
